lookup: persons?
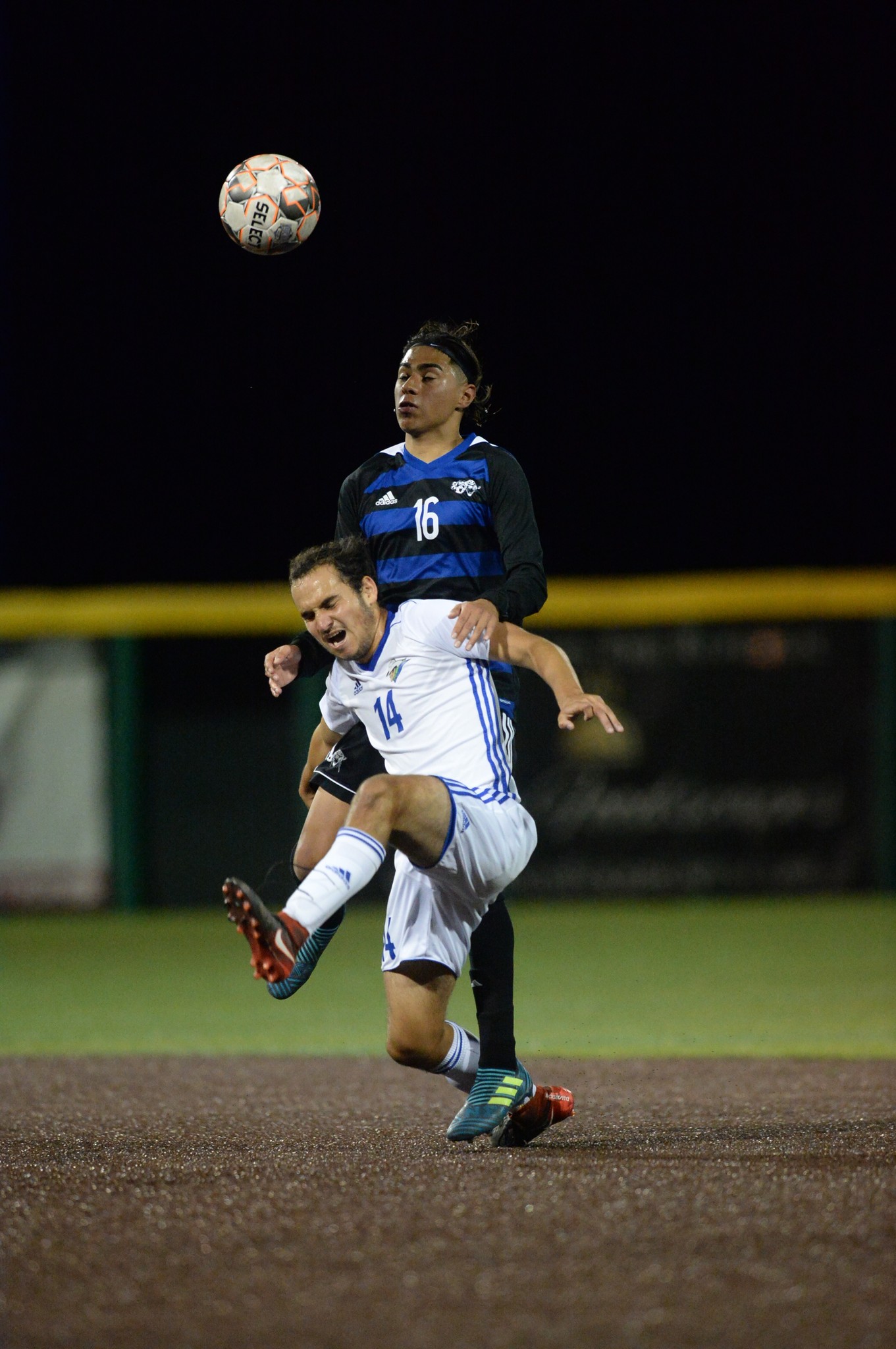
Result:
[220,531,631,1154]
[261,322,553,1146]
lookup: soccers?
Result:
[219,154,321,255]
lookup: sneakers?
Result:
[267,923,343,999]
[223,877,295,984]
[446,1057,536,1143]
[493,1086,576,1148]
